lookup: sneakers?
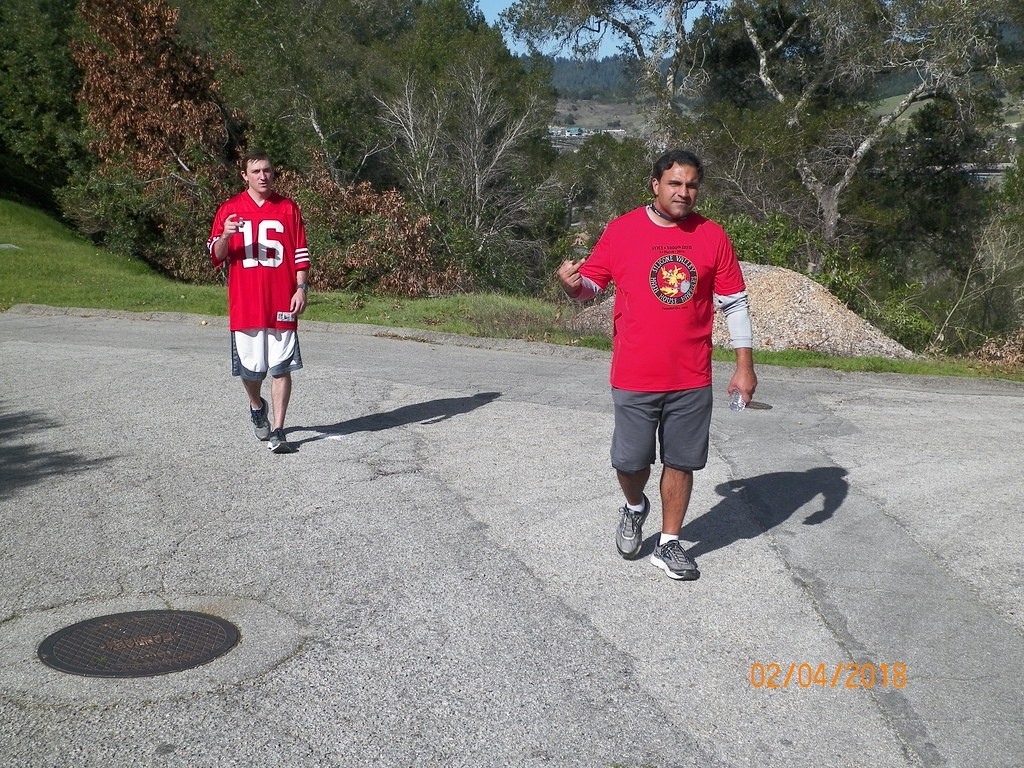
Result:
[651,540,698,580]
[267,428,291,452]
[616,493,651,558]
[250,397,271,441]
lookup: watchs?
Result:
[296,284,308,292]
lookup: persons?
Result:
[557,150,757,580]
[207,150,312,452]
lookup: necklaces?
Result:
[649,203,690,222]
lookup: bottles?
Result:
[729,389,747,411]
